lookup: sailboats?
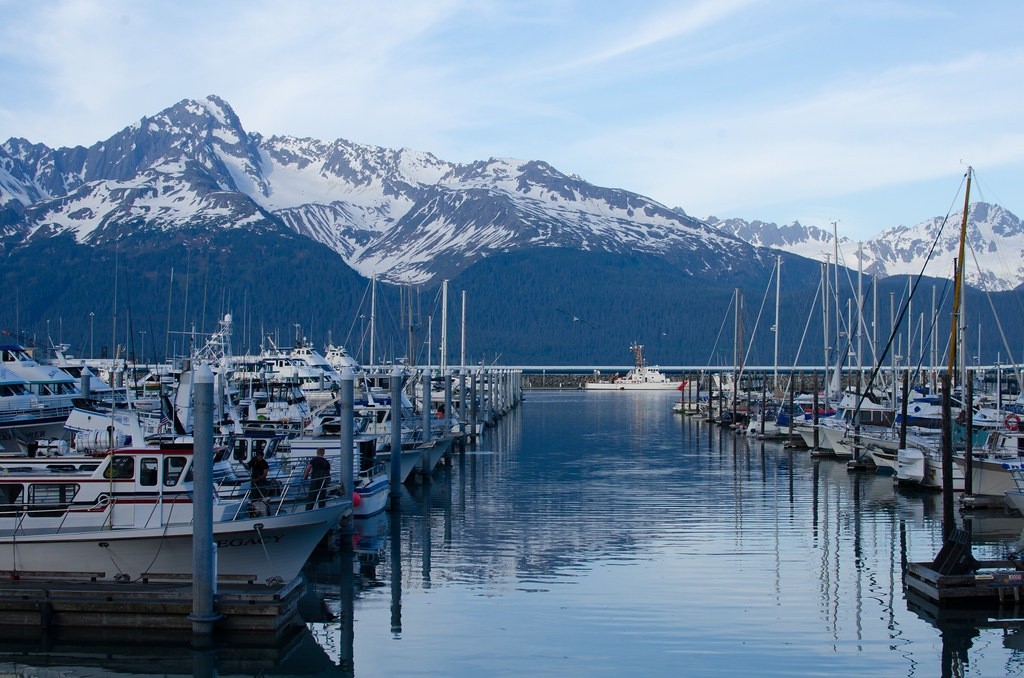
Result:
[671,165,1024,529]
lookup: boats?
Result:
[1,235,525,591]
[584,339,689,391]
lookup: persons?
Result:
[304,448,330,512]
[239,451,274,517]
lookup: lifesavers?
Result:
[1004,414,1020,431]
